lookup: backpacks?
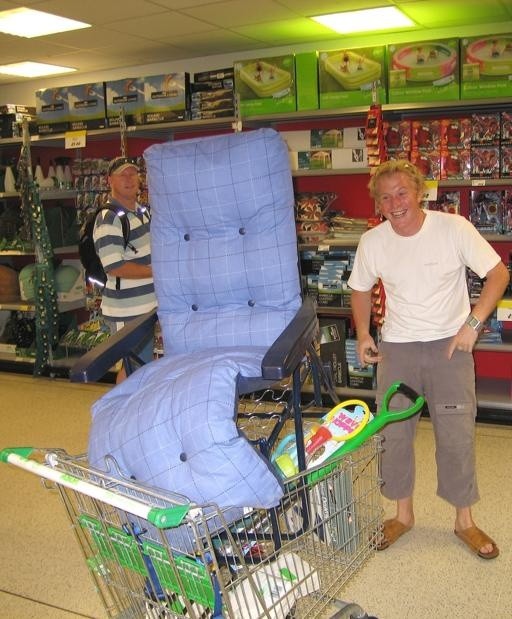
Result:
[79,204,131,292]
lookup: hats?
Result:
[107,156,140,175]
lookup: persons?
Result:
[346,157,510,562]
[92,156,157,385]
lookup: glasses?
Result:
[108,158,136,176]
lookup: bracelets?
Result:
[465,314,481,333]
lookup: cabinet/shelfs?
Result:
[1,97,512,409]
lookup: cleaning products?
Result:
[3,160,16,191]
[35,154,73,191]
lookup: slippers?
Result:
[454,525,499,559]
[367,518,410,550]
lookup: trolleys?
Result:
[0,430,389,619]
